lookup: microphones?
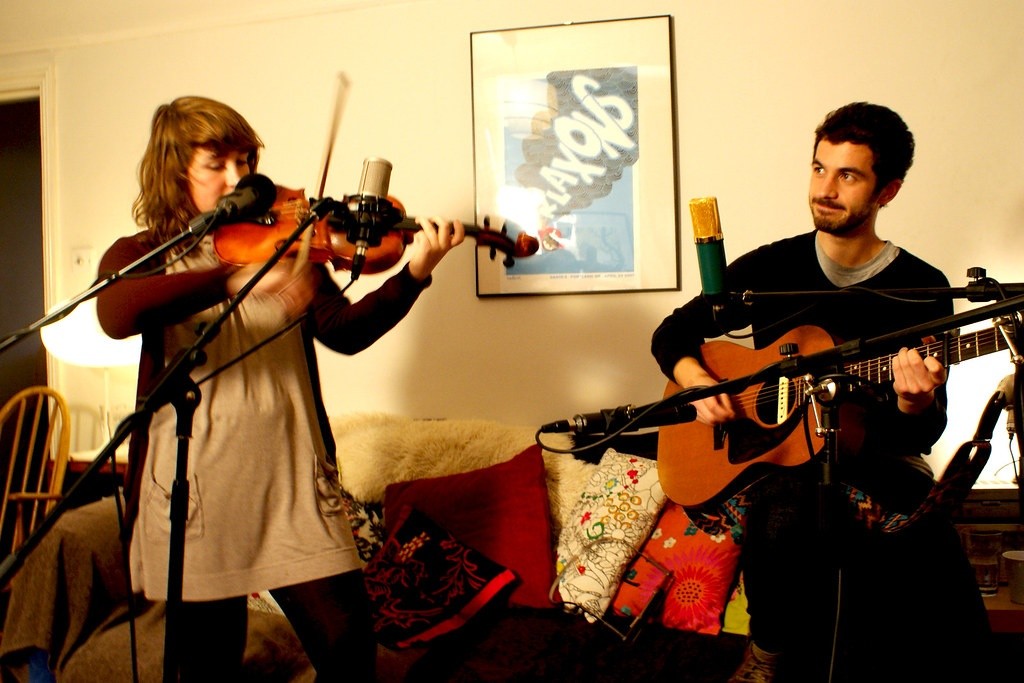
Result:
[544,403,699,442]
[349,156,392,281]
[190,173,276,233]
[690,196,727,321]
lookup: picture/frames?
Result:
[470,15,681,299]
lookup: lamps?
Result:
[39,295,145,462]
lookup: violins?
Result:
[211,181,541,269]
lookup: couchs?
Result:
[0,413,753,683]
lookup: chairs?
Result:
[2,387,73,565]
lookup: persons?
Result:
[96,96,468,682]
[651,100,959,681]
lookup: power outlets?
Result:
[70,247,95,273]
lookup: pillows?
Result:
[357,499,524,649]
[383,443,564,608]
[551,448,669,626]
[609,488,752,637]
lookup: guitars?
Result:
[655,302,1023,514]
